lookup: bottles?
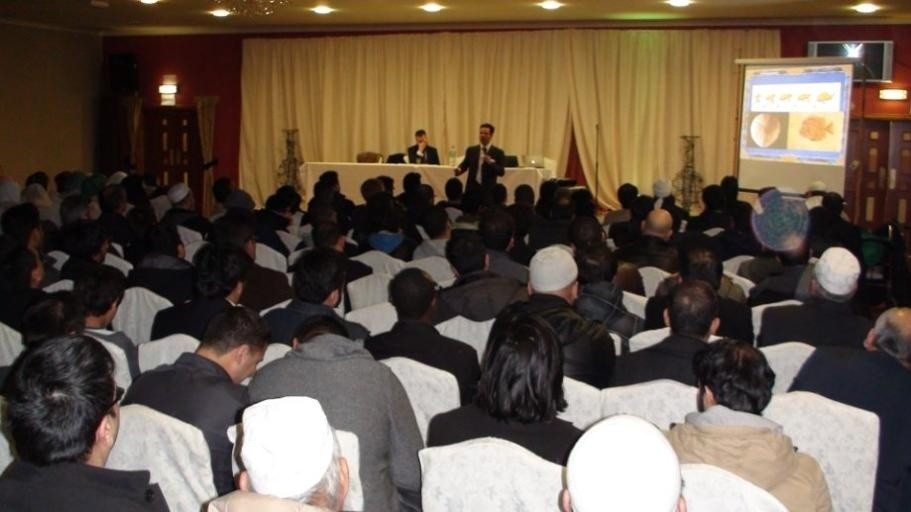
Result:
[449,145,457,166]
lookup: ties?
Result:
[481,146,486,155]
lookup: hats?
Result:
[167,182,191,204]
[527,246,580,294]
[240,394,336,504]
[809,180,827,193]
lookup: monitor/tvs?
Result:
[807,40,894,83]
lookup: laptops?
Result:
[522,154,547,168]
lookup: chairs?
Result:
[356,152,385,164]
[386,153,411,163]
[503,155,518,167]
[0,195,911,512]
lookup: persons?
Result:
[198,397,351,511]
[555,411,687,510]
[661,341,835,511]
[246,316,424,511]
[454,123,505,192]
[0,333,168,512]
[118,310,269,498]
[788,307,911,510]
[428,316,584,469]
[408,129,440,165]
[1,170,911,408]
[454,123,505,206]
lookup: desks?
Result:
[299,160,551,207]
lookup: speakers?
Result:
[109,50,139,92]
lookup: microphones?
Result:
[482,146,488,166]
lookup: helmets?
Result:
[813,245,862,296]
